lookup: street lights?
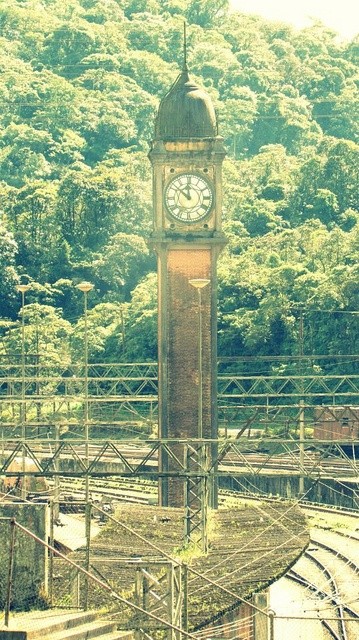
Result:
[188,278,212,554]
[15,285,31,499]
[76,281,94,502]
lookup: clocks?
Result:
[165,174,213,222]
